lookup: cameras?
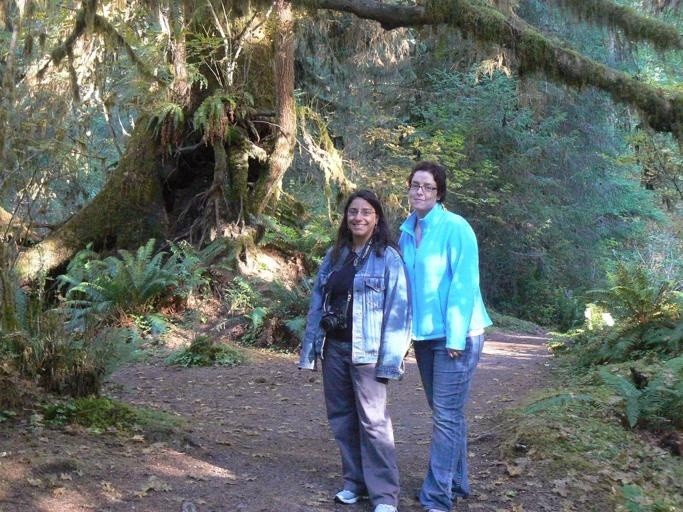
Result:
[319,307,347,331]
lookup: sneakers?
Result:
[374,504,398,512]
[333,491,369,504]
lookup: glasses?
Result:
[408,183,437,191]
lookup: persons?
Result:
[398,161,493,512]
[298,190,413,512]
